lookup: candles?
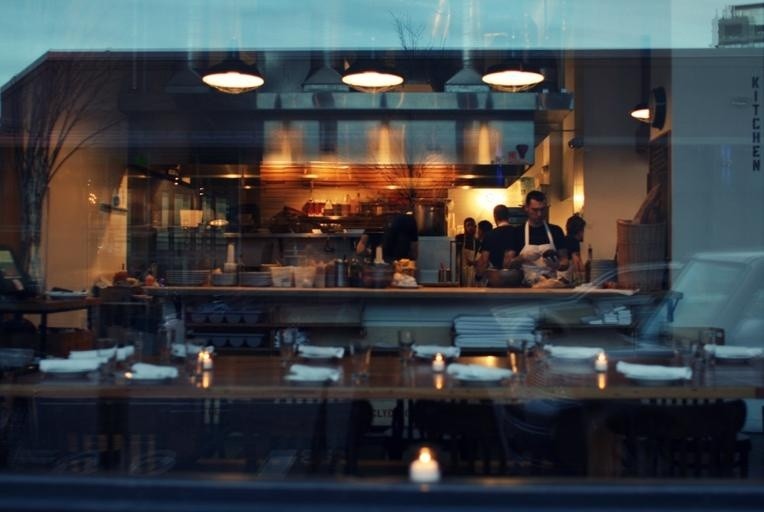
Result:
[431,351,445,372]
[594,351,608,372]
[197,352,213,367]
[406,447,444,483]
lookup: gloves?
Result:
[519,244,542,264]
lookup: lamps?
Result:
[199,52,268,95]
[482,49,548,90]
[339,50,406,94]
[628,100,651,120]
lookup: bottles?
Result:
[326,264,336,287]
[325,200,333,216]
[316,261,326,288]
[334,260,345,287]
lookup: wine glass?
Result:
[398,327,417,362]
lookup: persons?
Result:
[455,217,479,266]
[503,191,569,277]
[379,213,419,258]
[474,205,523,280]
[357,223,388,254]
[477,221,492,247]
[560,216,586,255]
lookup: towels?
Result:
[704,342,760,361]
[69,345,119,361]
[543,340,604,361]
[131,359,179,380]
[37,357,107,374]
[447,363,515,383]
[296,342,346,360]
[454,314,538,350]
[285,361,340,381]
[614,359,693,382]
[410,341,462,361]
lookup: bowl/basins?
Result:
[273,265,316,288]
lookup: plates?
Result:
[549,352,594,363]
[40,367,98,376]
[237,272,272,287]
[124,371,171,384]
[451,372,507,386]
[623,373,684,386]
[413,349,452,359]
[297,352,336,359]
[711,348,753,362]
[284,373,329,385]
[164,268,211,286]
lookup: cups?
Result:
[696,329,716,374]
[95,337,118,377]
[349,339,370,377]
[505,337,529,379]
